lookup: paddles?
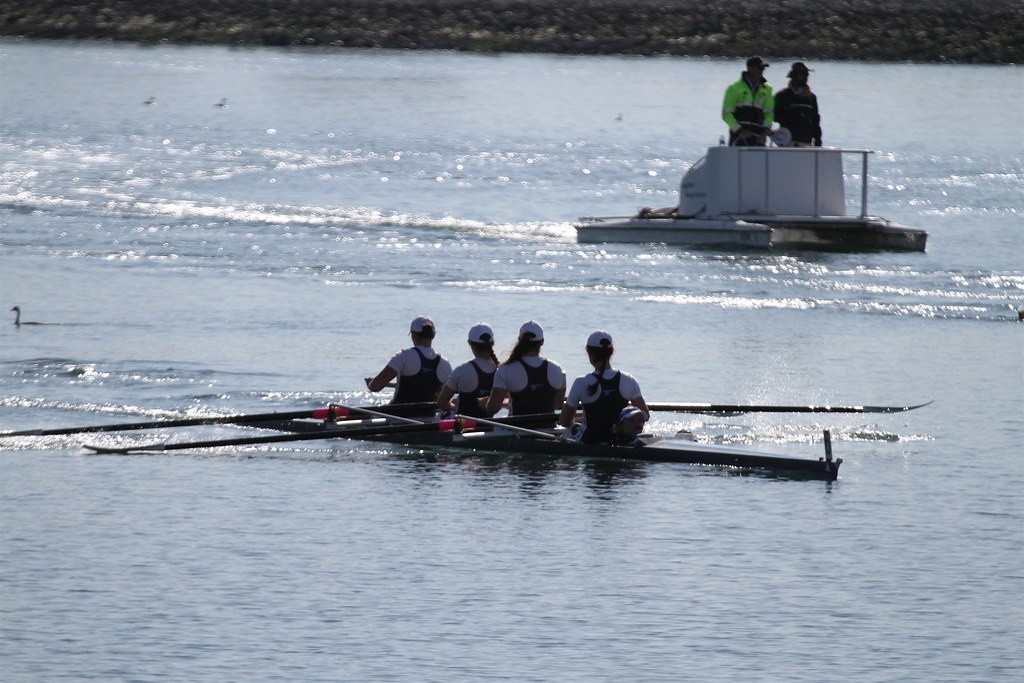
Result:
[1,401,437,439]
[645,400,936,415]
[83,413,563,454]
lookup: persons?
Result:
[773,62,822,146]
[559,331,650,444]
[478,320,567,429]
[437,322,500,419]
[722,57,774,146]
[364,314,453,416]
[611,406,648,446]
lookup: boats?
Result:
[570,125,931,254]
[233,408,843,482]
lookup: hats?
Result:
[746,56,769,75]
[587,330,613,348]
[409,316,436,334]
[468,321,495,343]
[519,320,544,341]
[787,61,812,77]
